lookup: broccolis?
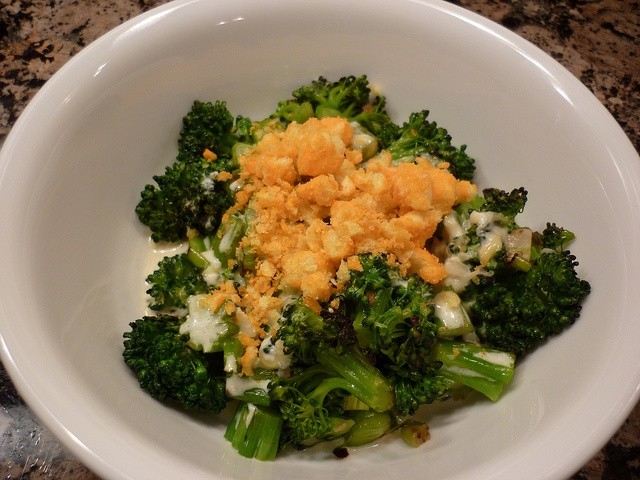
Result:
[123,76,590,463]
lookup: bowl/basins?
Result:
[0,1,640,480]
